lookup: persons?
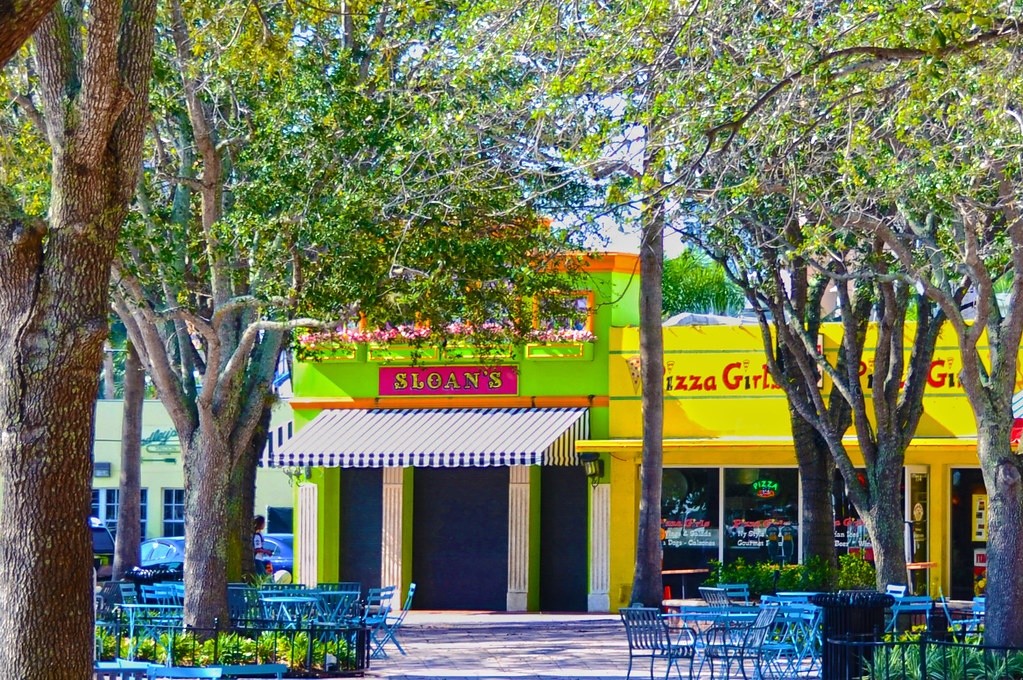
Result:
[253,515,271,575]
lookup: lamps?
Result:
[581,451,604,488]
[94,463,110,478]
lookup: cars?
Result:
[138,537,191,583]
[260,533,295,581]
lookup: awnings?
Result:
[255,422,293,468]
[1012,390,1023,419]
[272,408,589,466]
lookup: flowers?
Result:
[296,320,599,342]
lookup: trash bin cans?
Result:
[807,590,895,680]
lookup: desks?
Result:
[661,569,709,599]
[778,591,828,596]
[656,613,758,680]
[260,596,318,630]
[117,603,185,668]
[310,590,361,641]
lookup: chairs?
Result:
[96,580,416,659]
[620,583,985,680]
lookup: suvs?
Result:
[91,517,116,582]
